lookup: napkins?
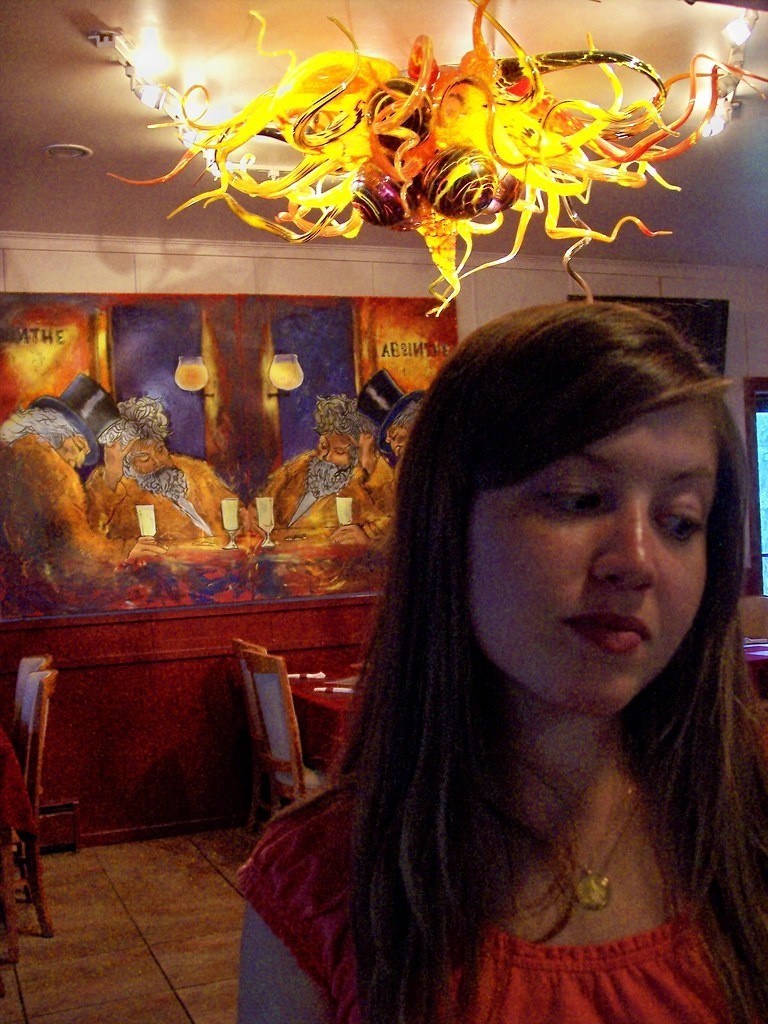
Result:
[287,673,326,679]
[314,687,352,693]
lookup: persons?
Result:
[232,301,767,1022]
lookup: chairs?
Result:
[231,637,326,831]
[0,654,63,937]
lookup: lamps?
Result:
[702,10,759,137]
[94,0,767,314]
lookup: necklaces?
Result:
[564,788,633,908]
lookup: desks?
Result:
[288,671,357,764]
